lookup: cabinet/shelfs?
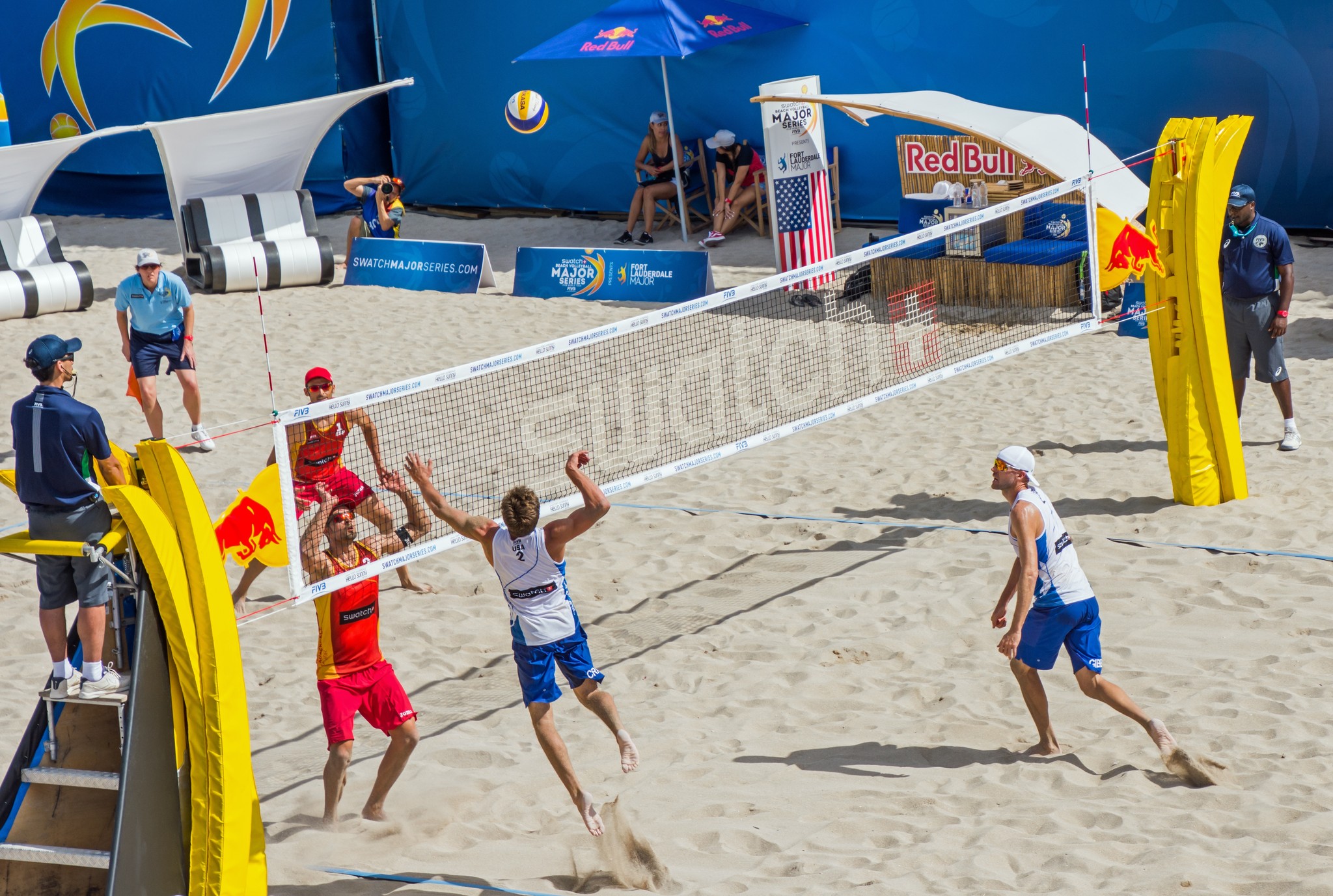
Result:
[943,202,1009,257]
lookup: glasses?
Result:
[1226,200,1254,210]
[306,382,333,392]
[140,264,159,270]
[328,508,356,524]
[52,352,75,363]
[994,458,1029,472]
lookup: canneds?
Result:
[965,187,972,204]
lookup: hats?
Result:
[137,248,160,266]
[333,497,357,511]
[650,110,669,124]
[1228,184,1256,207]
[997,445,1040,486]
[305,367,333,387]
[706,129,735,149]
[26,334,82,371]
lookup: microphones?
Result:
[60,363,76,376]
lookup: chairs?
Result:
[826,142,843,235]
[984,202,1089,268]
[632,137,711,238]
[862,197,955,259]
[720,138,765,239]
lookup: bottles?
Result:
[972,183,980,207]
[953,182,962,207]
[980,181,988,206]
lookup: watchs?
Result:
[1276,310,1289,318]
[725,198,732,203]
[184,336,194,341]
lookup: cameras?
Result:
[380,182,394,194]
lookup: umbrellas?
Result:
[511,0,809,244]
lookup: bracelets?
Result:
[658,166,664,174]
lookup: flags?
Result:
[126,363,144,410]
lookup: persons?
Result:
[116,247,217,453]
[697,129,766,250]
[1218,184,1303,451]
[615,111,689,246]
[231,368,432,822]
[342,175,406,270]
[11,335,132,699]
[404,452,639,838]
[991,446,1179,755]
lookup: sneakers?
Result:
[635,231,653,245]
[79,661,132,699]
[49,664,82,699]
[698,231,726,249]
[1280,426,1302,450]
[613,230,632,245]
[191,426,215,451]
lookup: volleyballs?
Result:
[504,90,549,134]
